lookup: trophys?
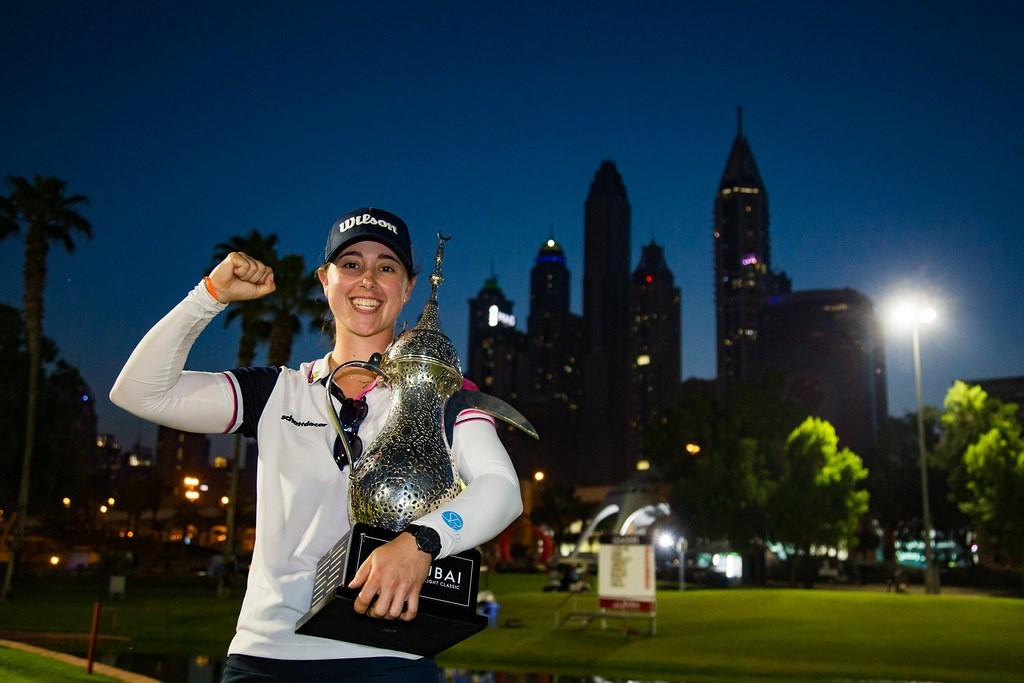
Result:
[293,229,542,659]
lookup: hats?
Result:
[322,207,412,275]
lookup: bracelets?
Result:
[204,275,218,301]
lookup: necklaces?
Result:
[329,356,374,387]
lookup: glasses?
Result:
[333,399,371,470]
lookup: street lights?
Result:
[886,303,942,592]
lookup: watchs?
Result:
[403,524,443,566]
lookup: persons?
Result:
[562,562,591,593]
[109,208,525,683]
